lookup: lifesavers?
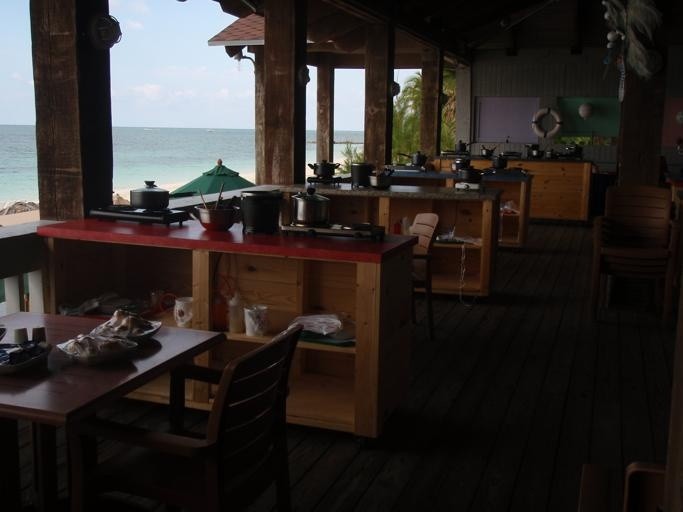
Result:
[532,108,561,138]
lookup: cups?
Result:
[32,327,46,341]
[173,297,192,328]
[244,304,269,336]
[12,328,28,344]
[546,152,552,159]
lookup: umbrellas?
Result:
[170,160,255,196]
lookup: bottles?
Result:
[213,292,226,330]
[229,288,243,332]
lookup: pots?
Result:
[130,139,545,227]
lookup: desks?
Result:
[0,311,227,510]
[430,154,591,223]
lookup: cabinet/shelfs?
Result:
[256,185,503,299]
[36,219,418,439]
[385,168,535,250]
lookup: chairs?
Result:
[84,320,304,509]
[584,181,681,329]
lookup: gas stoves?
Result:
[89,205,188,225]
[281,224,385,242]
